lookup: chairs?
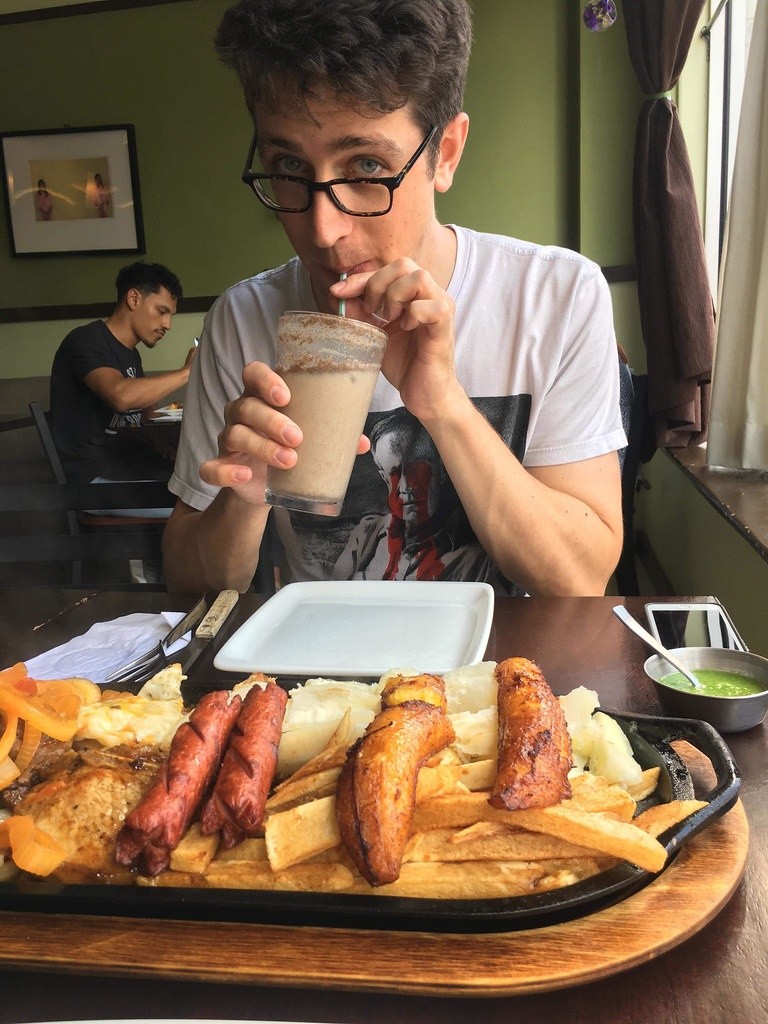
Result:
[28,401,175,586]
[0,481,177,592]
[613,374,647,596]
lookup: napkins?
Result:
[23,611,192,684]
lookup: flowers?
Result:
[582,0,617,33]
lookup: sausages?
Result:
[115,682,289,874]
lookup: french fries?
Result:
[139,707,712,901]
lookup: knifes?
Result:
[172,590,238,674]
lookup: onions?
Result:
[0,661,81,877]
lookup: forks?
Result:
[104,591,208,681]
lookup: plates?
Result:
[214,579,495,678]
[1,682,742,935]
[154,404,184,416]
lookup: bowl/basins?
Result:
[645,648,767,733]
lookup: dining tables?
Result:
[0,591,768,1024]
[118,423,182,446]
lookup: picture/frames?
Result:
[0,123,147,260]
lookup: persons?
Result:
[50,262,196,518]
[160,0,628,597]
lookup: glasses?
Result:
[242,123,437,217]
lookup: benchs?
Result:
[0,372,188,579]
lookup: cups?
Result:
[262,311,386,517]
